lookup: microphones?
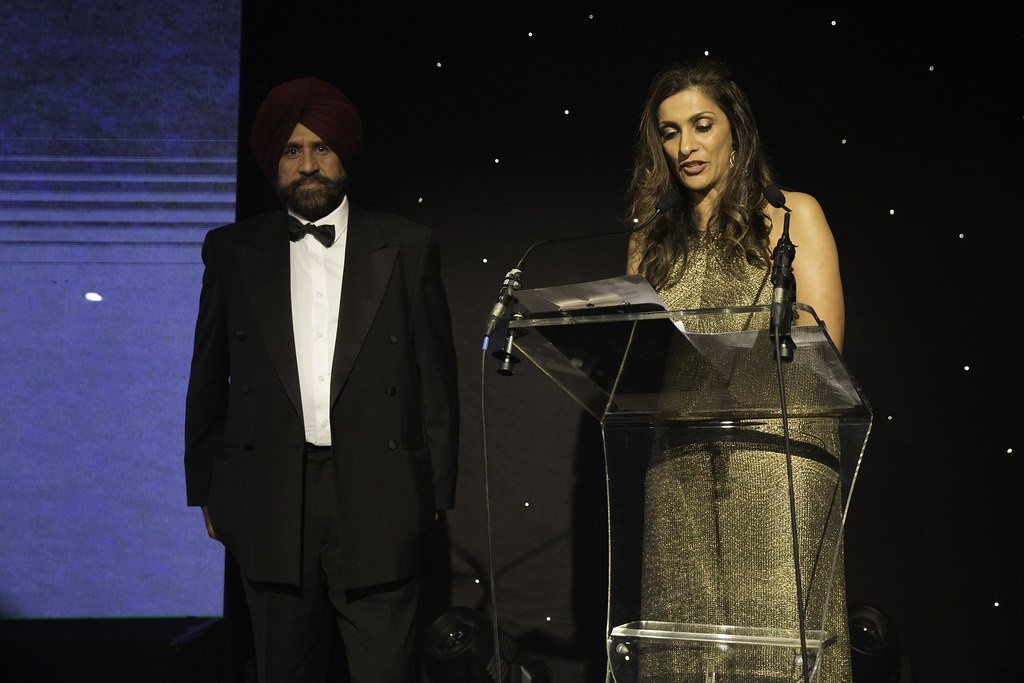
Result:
[762,185,808,327]
[482,189,677,339]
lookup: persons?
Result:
[626,56,855,683]
[185,77,460,683]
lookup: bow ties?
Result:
[288,216,335,247]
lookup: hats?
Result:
[251,78,363,187]
